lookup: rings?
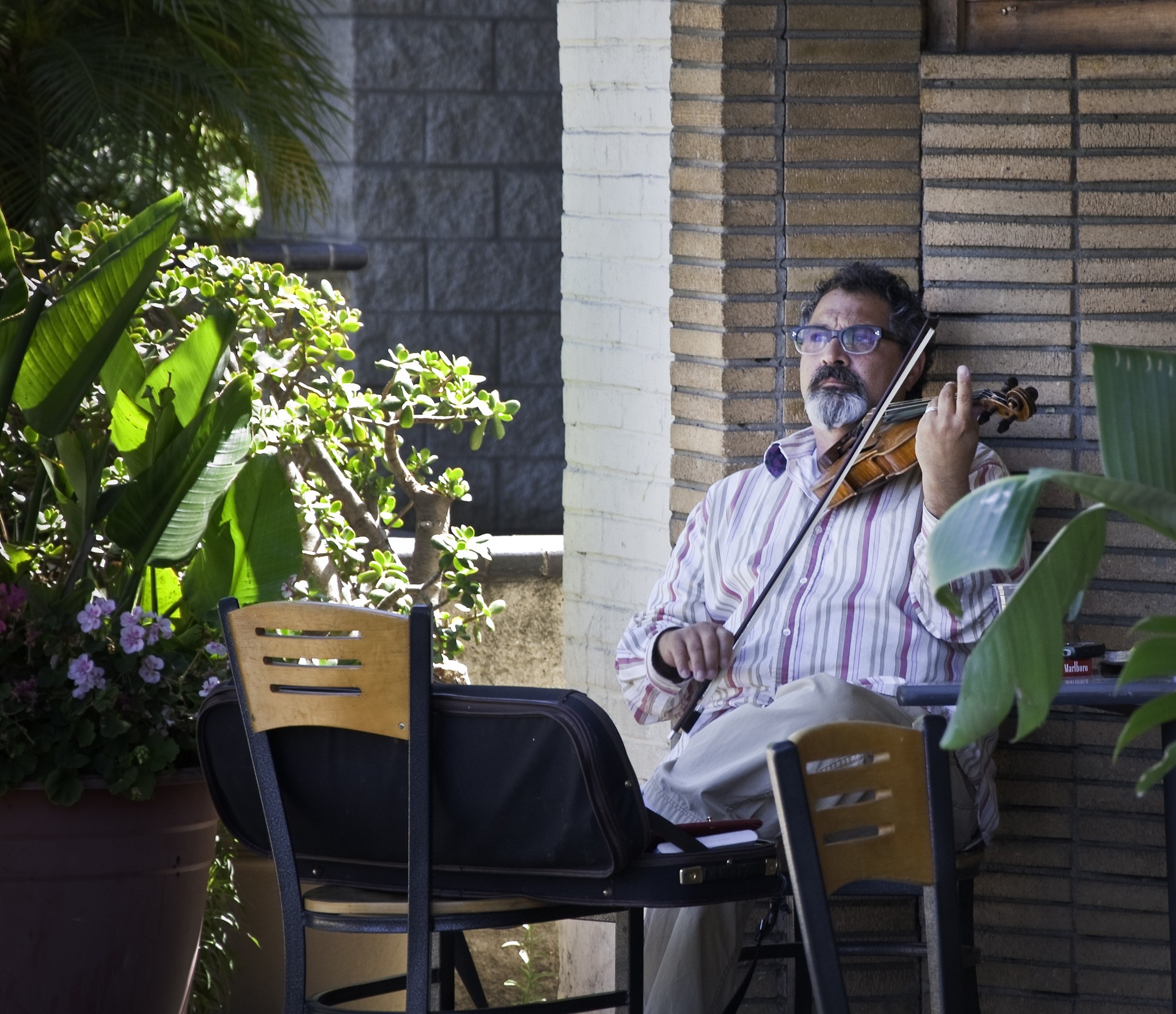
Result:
[924,406,936,413]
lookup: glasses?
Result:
[791,324,908,355]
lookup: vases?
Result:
[0,767,219,1014]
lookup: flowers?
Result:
[0,583,225,717]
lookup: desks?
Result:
[897,679,1176,1014]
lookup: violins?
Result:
[810,376,1039,509]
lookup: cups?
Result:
[991,583,1019,613]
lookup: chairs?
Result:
[216,597,645,1014]
[766,715,981,1014]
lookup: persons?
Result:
[613,263,1030,1014]
